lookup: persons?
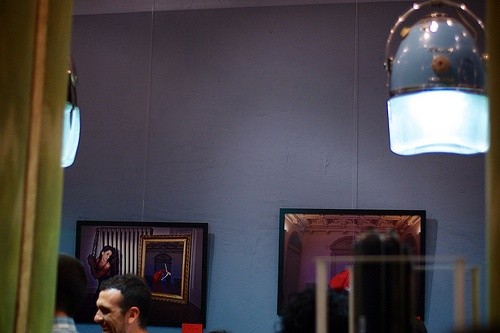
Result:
[366,308,427,333]
[51,254,87,333]
[93,273,152,333]
[273,285,361,333]
[88,245,116,280]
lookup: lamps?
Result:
[385,0,492,156]
[61,66,81,169]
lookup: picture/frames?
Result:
[277,209,427,316]
[75,221,210,329]
